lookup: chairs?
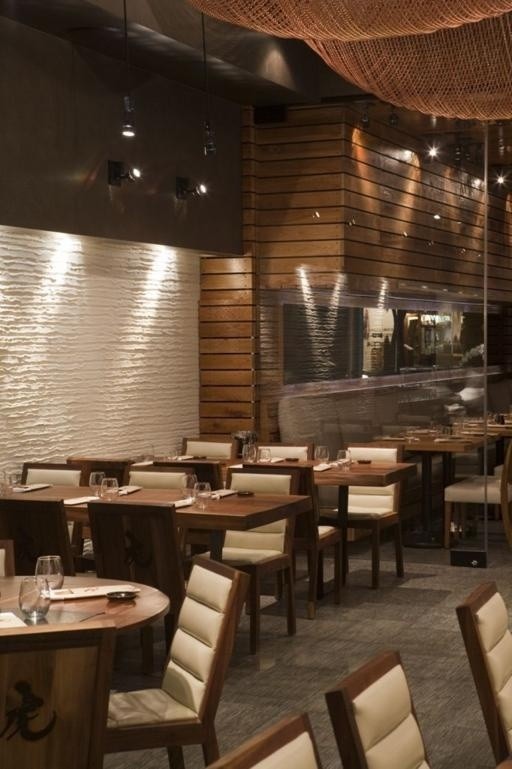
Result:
[317,442,404,587]
[67,456,134,490]
[444,440,512,549]
[206,713,325,768]
[152,459,225,493]
[324,648,431,769]
[87,501,189,652]
[101,551,251,769]
[242,462,341,620]
[254,440,314,463]
[181,437,239,463]
[0,495,94,577]
[196,468,302,647]
[450,578,512,769]
[21,461,91,570]
[126,468,192,492]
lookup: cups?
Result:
[242,444,257,465]
[18,576,50,619]
[336,450,352,471]
[143,445,154,462]
[89,471,105,497]
[258,448,271,463]
[166,448,177,461]
[193,483,211,509]
[5,468,22,488]
[35,556,64,591]
[314,446,329,463]
[178,474,198,499]
[100,478,119,500]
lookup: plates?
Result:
[236,492,255,496]
[285,458,299,461]
[357,458,372,464]
[105,592,138,602]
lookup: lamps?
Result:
[176,176,206,203]
[198,123,217,158]
[110,156,142,187]
[119,118,138,139]
